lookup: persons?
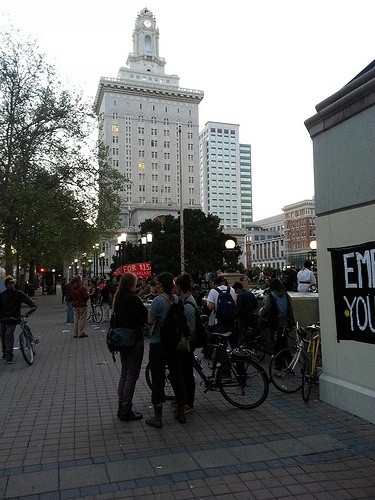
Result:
[145,271,186,430]
[206,270,247,390]
[0,274,37,365]
[254,279,296,373]
[296,260,317,293]
[60,275,215,339]
[232,282,260,346]
[175,273,198,413]
[114,273,149,422]
[259,276,271,286]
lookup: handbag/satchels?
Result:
[239,312,258,337]
[106,328,139,353]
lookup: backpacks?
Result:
[159,294,191,358]
[214,286,236,322]
[184,302,210,347]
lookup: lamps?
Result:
[144,19,152,27]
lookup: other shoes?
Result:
[7,360,13,364]
[79,334,88,338]
[73,335,78,338]
[1,356,7,360]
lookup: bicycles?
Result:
[86,297,103,323]
[16,310,40,365]
[145,320,323,409]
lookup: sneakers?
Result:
[273,371,282,378]
[175,413,186,423]
[145,416,163,428]
[285,370,294,376]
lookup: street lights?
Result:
[309,240,316,273]
[68,231,153,278]
[225,240,236,273]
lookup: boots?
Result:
[120,402,143,421]
[117,400,141,418]
